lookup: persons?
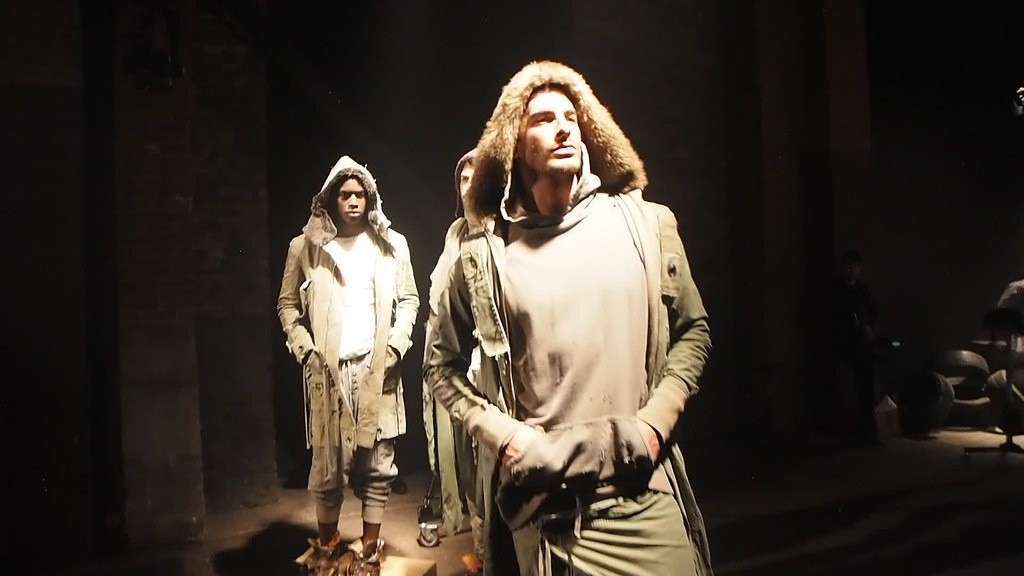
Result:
[421,60,713,576]
[277,156,420,576]
[406,149,485,562]
[809,249,878,433]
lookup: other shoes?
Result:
[347,537,386,576]
[391,475,408,495]
[304,529,341,576]
